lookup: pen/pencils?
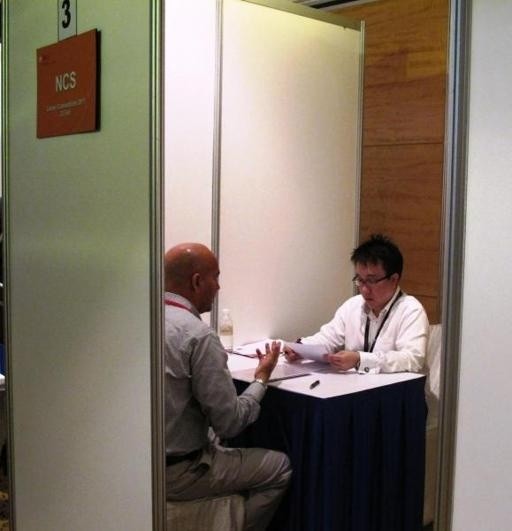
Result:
[282,338,301,356]
[310,381,319,389]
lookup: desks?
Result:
[227,337,427,531]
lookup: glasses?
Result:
[351,274,390,287]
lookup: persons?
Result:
[283,234,429,373]
[164,243,293,531]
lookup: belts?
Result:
[166,448,203,466]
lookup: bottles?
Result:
[219,308,233,355]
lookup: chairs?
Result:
[423,323,442,527]
[165,492,246,530]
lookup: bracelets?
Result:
[252,379,268,393]
